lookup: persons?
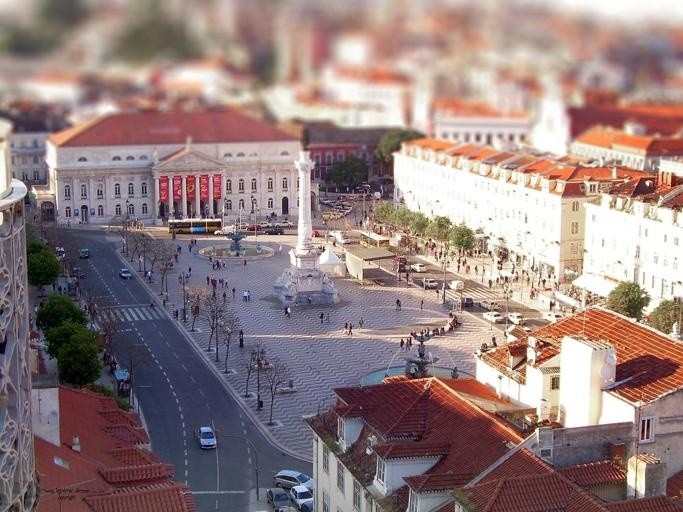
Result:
[259,358,269,369]
[145,237,251,310]
[359,196,609,350]
[344,322,352,336]
[58,286,66,295]
[284,305,291,318]
[308,296,313,303]
[320,312,330,324]
[295,303,299,307]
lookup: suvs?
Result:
[55,246,65,259]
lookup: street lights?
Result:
[208,302,227,362]
[250,193,254,213]
[176,270,191,324]
[125,199,130,219]
[128,340,154,428]
[250,344,269,412]
[170,208,175,239]
[221,435,261,500]
[252,199,258,237]
[238,198,243,231]
[122,219,132,258]
[223,196,229,217]
[138,233,151,277]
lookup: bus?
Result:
[166,218,221,234]
[359,231,390,253]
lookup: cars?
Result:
[317,184,382,222]
[542,311,563,324]
[267,468,314,511]
[213,220,294,234]
[328,230,343,236]
[118,268,131,280]
[78,249,89,260]
[199,425,217,452]
[70,267,87,279]
[337,235,350,244]
[420,278,437,289]
[460,297,532,335]
[411,263,426,273]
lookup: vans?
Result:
[447,281,464,291]
